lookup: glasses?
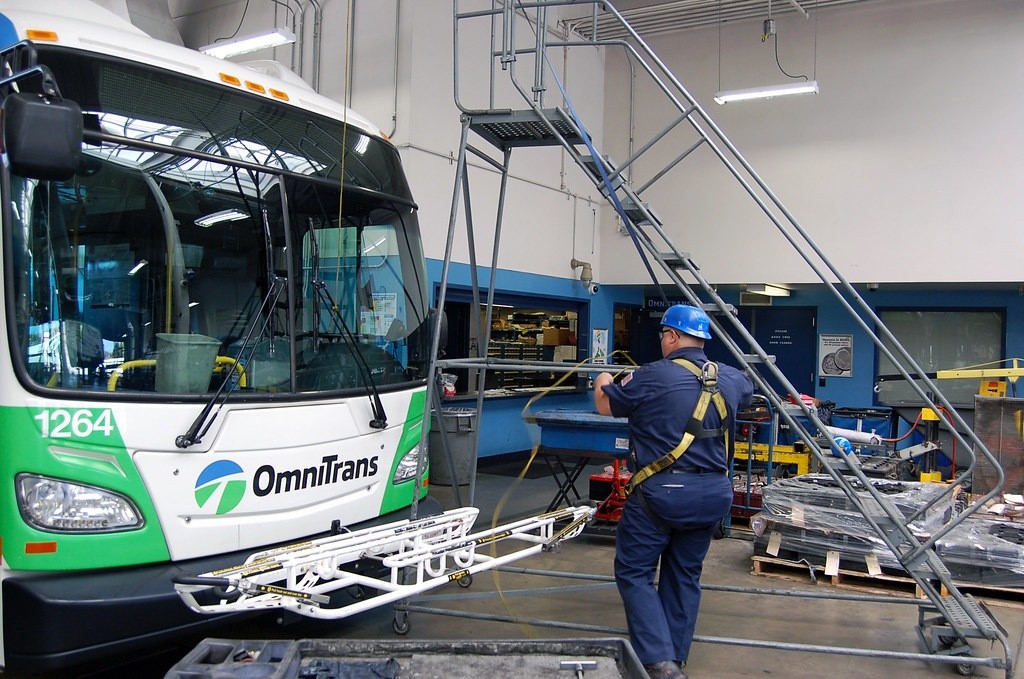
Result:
[659,329,680,340]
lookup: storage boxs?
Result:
[544,329,571,345]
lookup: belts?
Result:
[657,464,728,474]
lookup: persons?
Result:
[595,305,754,679]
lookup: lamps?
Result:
[714,0,819,105]
[747,285,790,296]
[199,0,296,59]
[571,258,593,289]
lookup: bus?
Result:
[0,0,597,671]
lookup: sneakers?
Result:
[646,660,691,679]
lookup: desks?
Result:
[536,410,636,513]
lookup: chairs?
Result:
[195,252,261,347]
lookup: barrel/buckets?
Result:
[429,407,477,486]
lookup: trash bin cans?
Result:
[154,333,222,395]
[427,406,478,486]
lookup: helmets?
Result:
[660,304,712,341]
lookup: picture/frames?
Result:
[818,334,853,378]
